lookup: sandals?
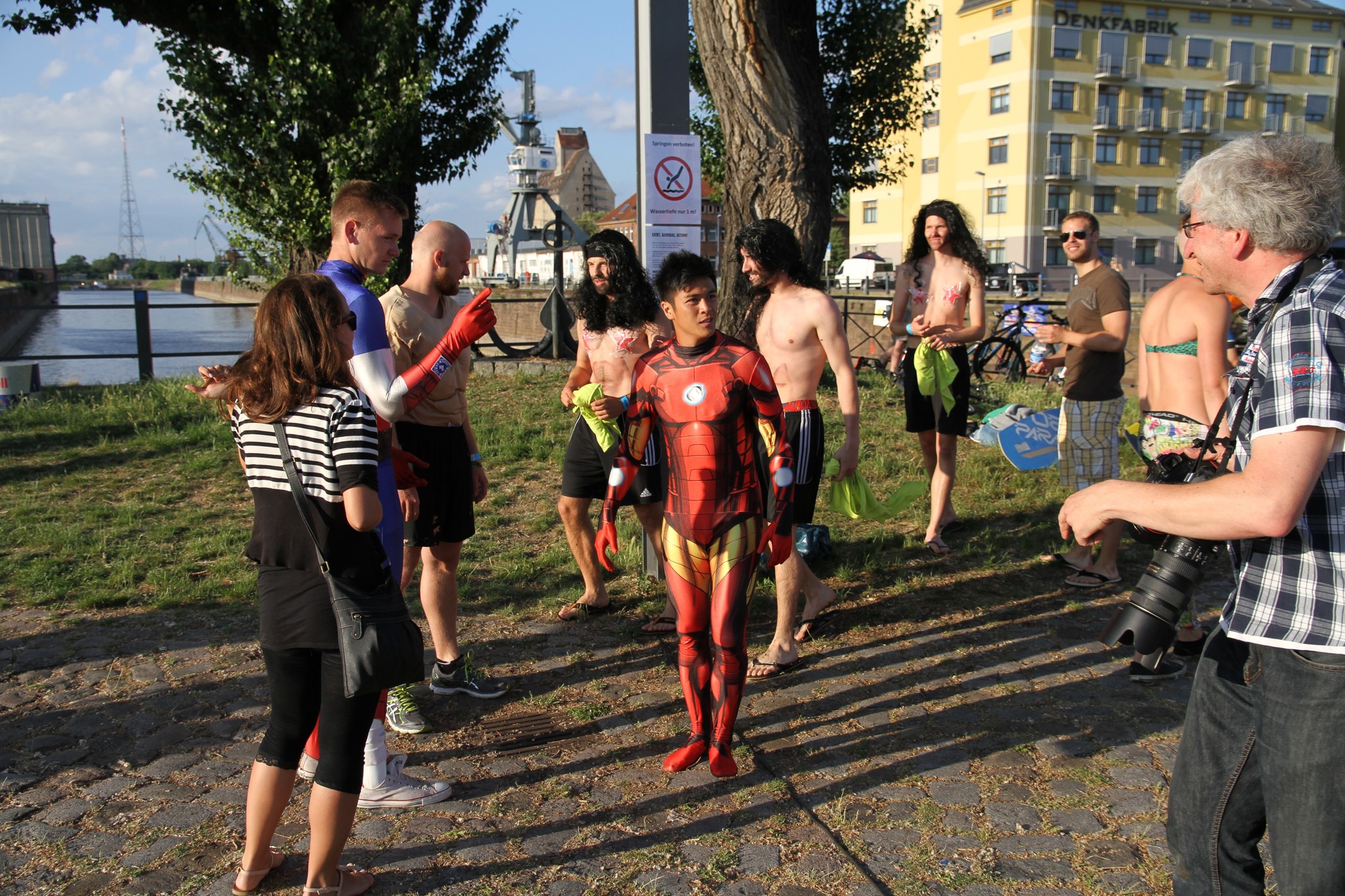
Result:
[232,846,286,896]
[302,864,377,896]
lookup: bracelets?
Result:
[905,323,916,335]
[472,462,482,467]
[620,395,632,413]
[471,452,482,460]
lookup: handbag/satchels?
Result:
[323,563,425,699]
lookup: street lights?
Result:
[974,169,985,258]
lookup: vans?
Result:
[833,257,897,289]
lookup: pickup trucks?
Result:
[983,262,1042,298]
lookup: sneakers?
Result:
[357,754,452,809]
[297,753,319,780]
[430,648,510,699]
[1172,631,1210,659]
[1128,658,1187,682]
[385,683,433,734]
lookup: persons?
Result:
[1057,130,1345,896]
[1024,211,1132,589]
[185,179,508,896]
[557,218,859,778]
[871,198,994,557]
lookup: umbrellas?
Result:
[852,250,886,276]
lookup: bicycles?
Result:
[892,298,1070,390]
[852,355,1007,417]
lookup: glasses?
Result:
[1059,230,1094,242]
[1182,215,1210,238]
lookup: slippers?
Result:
[926,541,950,556]
[794,609,845,643]
[924,521,962,537]
[1038,553,1082,571]
[1065,568,1122,587]
[639,615,676,635]
[557,599,612,621]
[746,657,806,680]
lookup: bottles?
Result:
[1029,337,1048,364]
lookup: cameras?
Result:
[1099,453,1244,672]
